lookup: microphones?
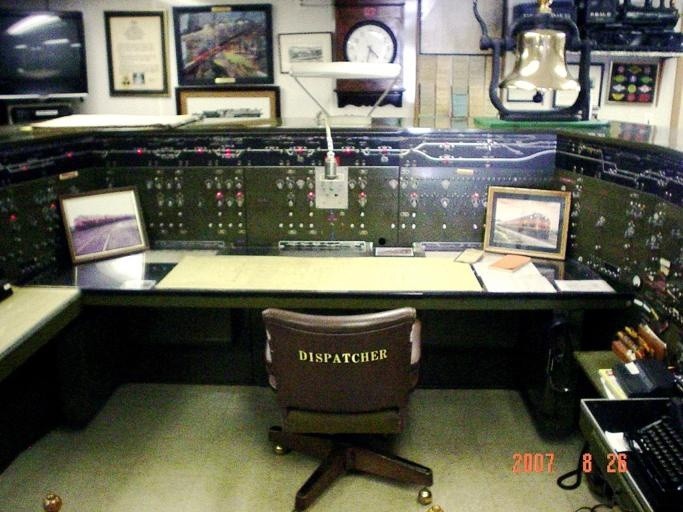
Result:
[325,151,337,179]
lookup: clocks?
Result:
[342,19,397,64]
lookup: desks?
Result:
[78,248,615,439]
[556,351,682,511]
[0,278,83,387]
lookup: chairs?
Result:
[261,304,434,511]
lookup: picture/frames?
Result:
[57,185,151,266]
[609,119,656,143]
[172,4,275,86]
[567,61,605,108]
[604,60,661,108]
[482,185,572,261]
[277,29,334,75]
[175,87,280,118]
[102,7,170,97]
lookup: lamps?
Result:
[287,61,403,177]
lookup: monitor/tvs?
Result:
[0,8,88,95]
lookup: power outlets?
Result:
[314,165,349,212]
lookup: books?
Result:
[31,112,204,132]
[489,254,531,273]
